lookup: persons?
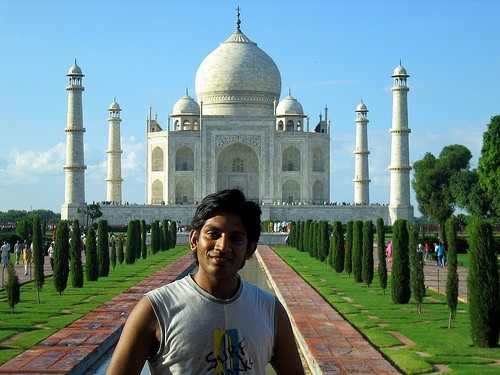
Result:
[269,220,291,233]
[4,240,10,266]
[0,241,9,269]
[14,241,23,264]
[22,244,32,275]
[48,243,55,270]
[21,239,29,265]
[80,231,86,257]
[159,219,187,232]
[417,241,447,268]
[386,240,393,263]
[105,188,306,375]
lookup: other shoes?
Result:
[435,263,438,266]
[24,273,27,275]
[440,264,443,268]
